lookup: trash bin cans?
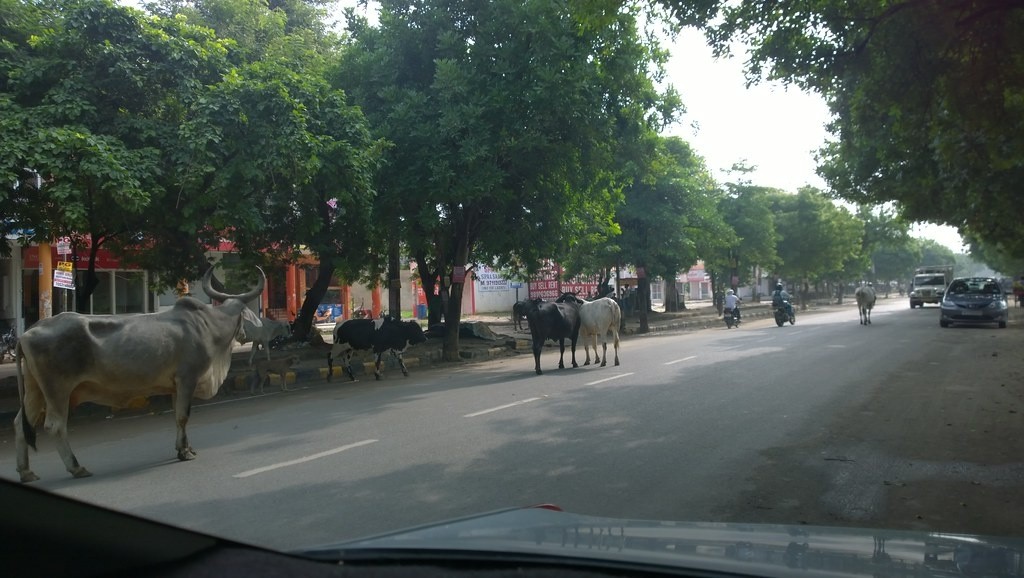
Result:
[416,304,427,320]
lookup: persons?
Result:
[586,283,631,302]
[772,283,792,318]
[724,290,741,320]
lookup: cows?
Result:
[11,264,266,485]
[510,287,621,376]
[854,285,877,325]
[327,315,427,384]
[235,316,292,372]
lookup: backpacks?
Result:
[772,290,785,307]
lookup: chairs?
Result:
[982,283,996,294]
[953,282,970,294]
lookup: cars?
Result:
[936,276,1011,328]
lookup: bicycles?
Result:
[0,326,17,361]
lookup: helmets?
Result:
[776,282,783,290]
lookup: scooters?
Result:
[724,298,743,329]
[311,304,333,325]
[774,295,796,327]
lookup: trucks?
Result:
[909,264,954,309]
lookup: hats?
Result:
[726,289,734,294]
